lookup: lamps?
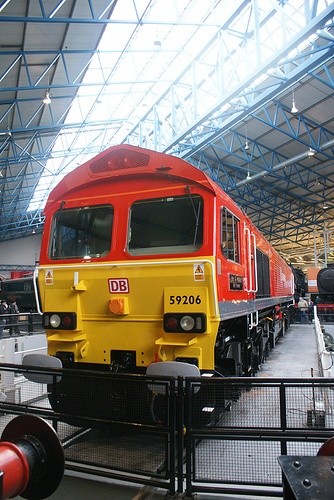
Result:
[308,148,314,156]
[32,214,42,233]
[6,113,12,137]
[290,89,299,113]
[43,72,51,104]
[323,203,328,209]
[246,159,252,180]
[245,124,250,150]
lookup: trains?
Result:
[0,269,42,311]
[34,143,308,435]
[317,262,334,321]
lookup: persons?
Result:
[314,296,322,304]
[297,297,308,324]
[7,299,24,337]
[307,298,314,322]
[0,299,9,339]
